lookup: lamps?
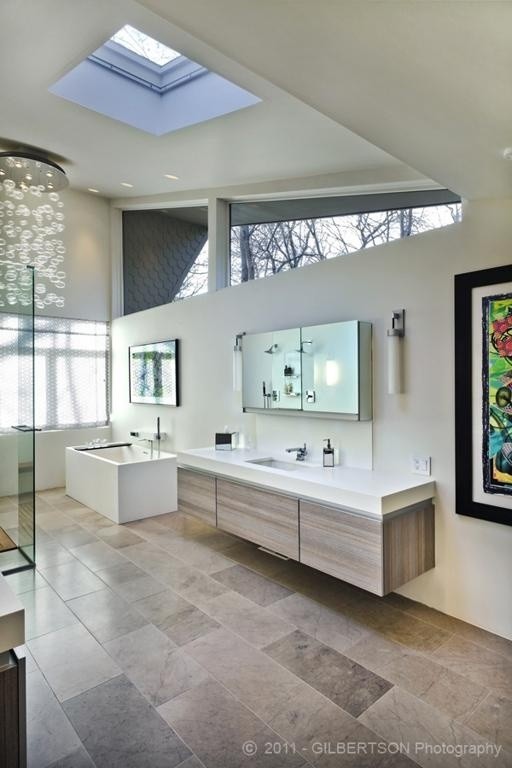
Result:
[384,308,406,396]
[231,331,248,391]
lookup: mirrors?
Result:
[242,320,360,414]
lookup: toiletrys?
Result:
[323,438,335,467]
[215,425,240,451]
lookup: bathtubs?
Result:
[64,441,178,526]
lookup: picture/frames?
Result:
[454,263,511,527]
[128,338,180,407]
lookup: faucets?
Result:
[137,439,153,459]
[285,442,307,462]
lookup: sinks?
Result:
[245,456,312,472]
